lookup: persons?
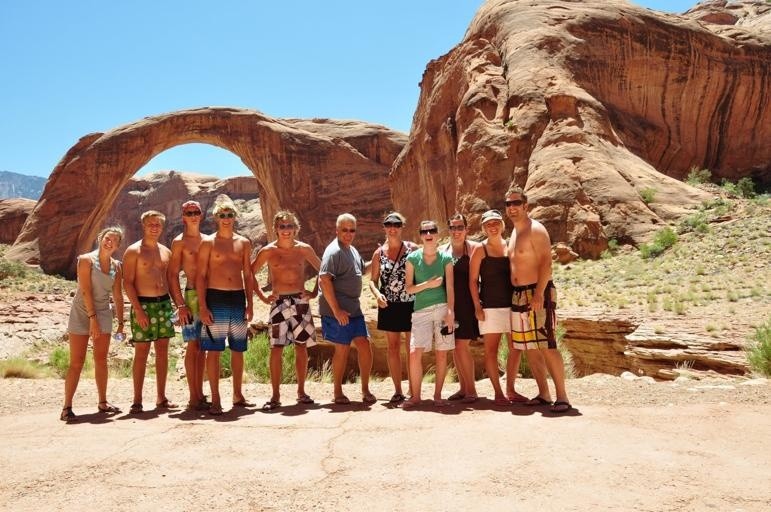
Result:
[504,188,571,411]
[251,211,321,412]
[167,200,212,411]
[60,227,123,421]
[400,220,455,409]
[469,210,529,406]
[437,213,480,403]
[319,214,380,405]
[123,210,179,415]
[369,212,420,402]
[196,201,256,415]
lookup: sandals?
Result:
[60,407,78,422]
[99,401,118,411]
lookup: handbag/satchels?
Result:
[376,243,404,294]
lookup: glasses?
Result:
[184,211,202,216]
[448,225,467,231]
[217,212,237,219]
[338,227,356,234]
[419,229,439,236]
[276,223,297,230]
[503,200,527,208]
[383,221,404,228]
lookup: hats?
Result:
[179,200,202,226]
[479,208,504,224]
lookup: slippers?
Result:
[295,396,314,404]
[155,399,179,408]
[263,401,281,411]
[210,405,223,416]
[460,396,480,404]
[448,394,464,400]
[508,394,529,404]
[129,404,144,413]
[526,397,554,406]
[396,401,424,408]
[492,397,512,407]
[331,395,351,404]
[362,393,377,403]
[233,398,256,408]
[434,399,444,408]
[203,398,213,406]
[188,402,210,410]
[551,401,572,413]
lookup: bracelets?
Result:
[88,313,97,318]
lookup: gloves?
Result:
[390,394,405,403]
